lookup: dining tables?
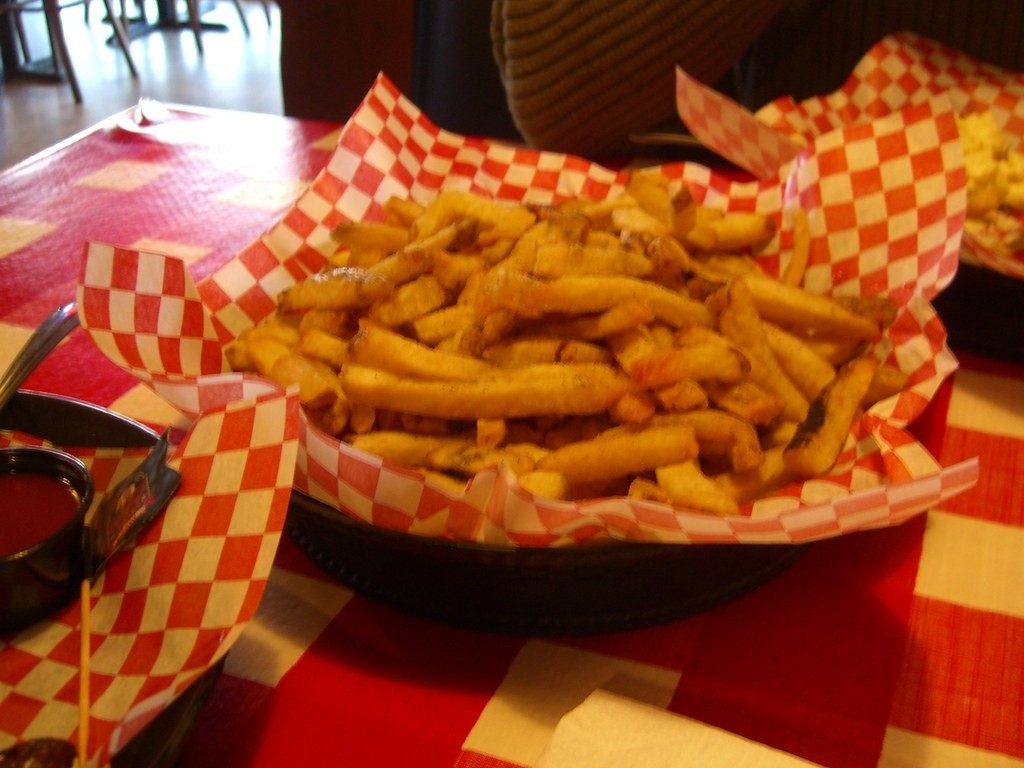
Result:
[0,99,1024,768]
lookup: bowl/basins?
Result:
[286,493,805,639]
[929,261,1024,365]
[0,387,230,768]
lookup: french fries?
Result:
[952,109,1024,257]
[224,178,900,514]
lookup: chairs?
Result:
[1,0,271,102]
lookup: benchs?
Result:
[408,0,1024,359]
